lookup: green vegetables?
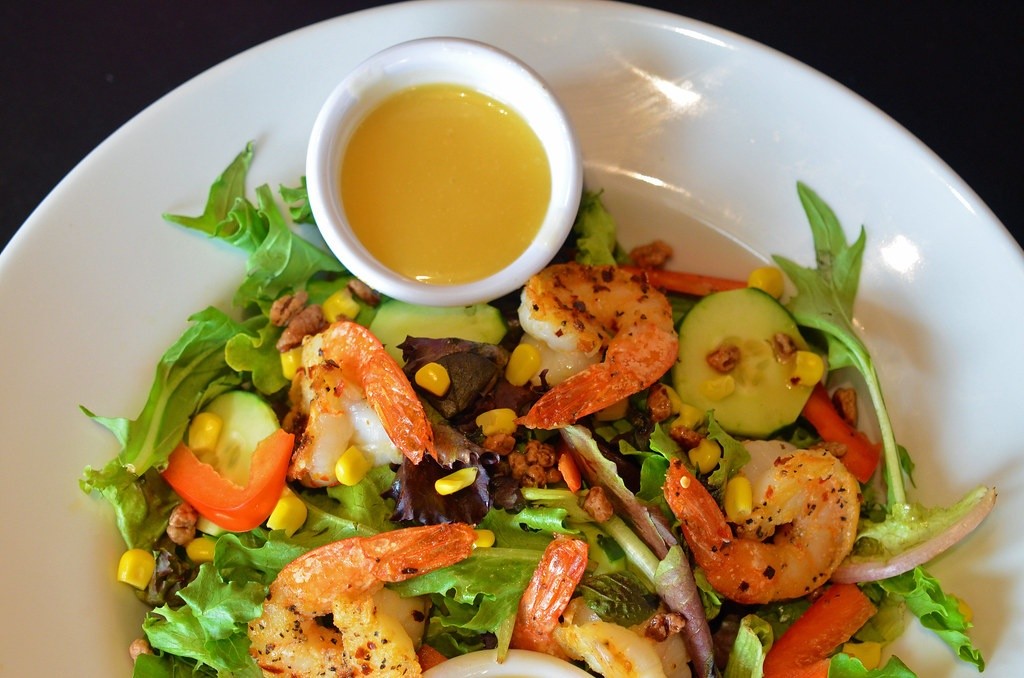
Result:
[77,137,998,678]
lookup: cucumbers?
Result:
[664,285,817,437]
[182,389,280,536]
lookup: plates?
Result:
[1,0,1024,677]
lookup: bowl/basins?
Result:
[306,38,583,308]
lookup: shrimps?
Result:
[659,436,867,605]
[285,320,434,489]
[517,534,694,678]
[245,520,478,678]
[514,262,679,431]
